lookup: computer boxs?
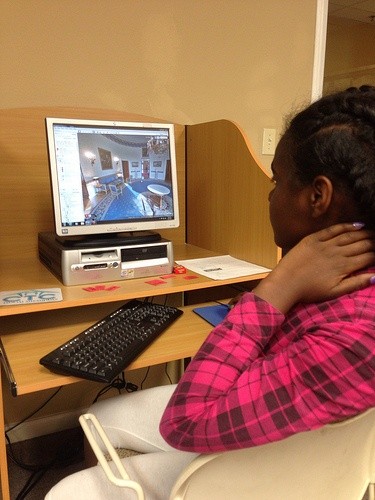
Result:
[38,231,174,286]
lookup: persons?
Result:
[45,85,375,500]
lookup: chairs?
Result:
[78,403,375,500]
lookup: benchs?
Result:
[92,171,122,194]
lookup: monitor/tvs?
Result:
[44,118,179,246]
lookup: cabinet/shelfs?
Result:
[0,106,283,500]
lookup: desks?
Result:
[109,181,123,198]
[147,184,171,210]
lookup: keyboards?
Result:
[39,299,182,384]
